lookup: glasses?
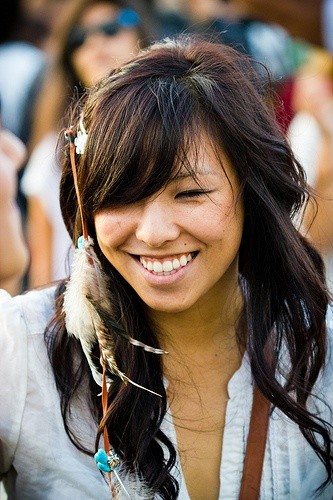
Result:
[69,20,127,49]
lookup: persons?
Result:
[0,1,332,296]
[0,36,333,500]
